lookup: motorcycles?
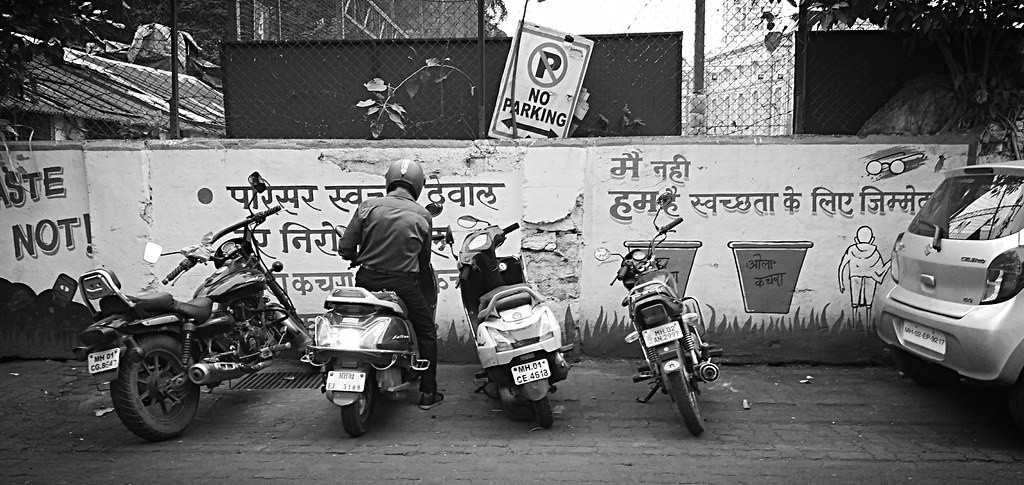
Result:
[594,192,723,436]
[71,171,313,442]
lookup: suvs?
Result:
[875,159,1024,447]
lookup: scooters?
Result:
[446,215,574,429]
[300,203,445,437]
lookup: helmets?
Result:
[385,159,424,201]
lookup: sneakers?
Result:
[420,392,445,410]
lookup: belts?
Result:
[361,264,416,277]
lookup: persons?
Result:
[337,157,446,410]
